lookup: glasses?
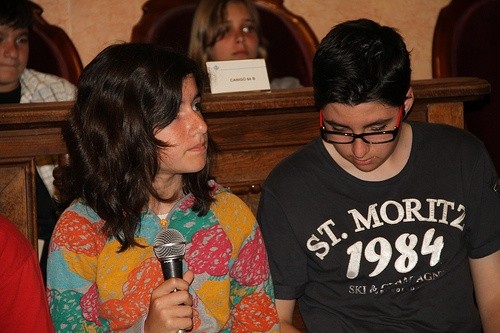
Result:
[319,104,402,144]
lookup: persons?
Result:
[0,0,78,104]
[256,18,500,333]
[188,0,300,90]
[47,43,280,332]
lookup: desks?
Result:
[0,78,492,294]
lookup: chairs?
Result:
[25,0,84,89]
[130,0,320,89]
[432,0,500,176]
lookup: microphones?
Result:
[153,229,186,333]
